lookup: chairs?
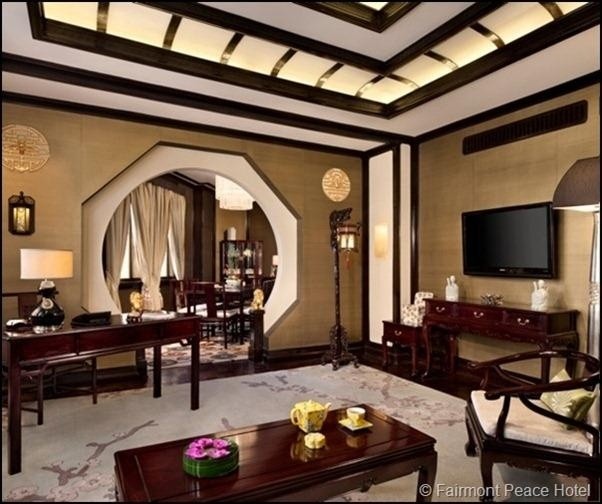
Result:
[169,232,280,351]
[0,286,102,425]
[458,347,601,503]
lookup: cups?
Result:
[347,407,366,425]
[347,434,368,448]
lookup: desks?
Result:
[111,399,437,503]
[1,307,205,475]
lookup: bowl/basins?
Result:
[180,437,239,479]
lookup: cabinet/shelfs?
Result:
[419,295,584,387]
[380,317,451,378]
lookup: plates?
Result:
[339,414,375,435]
[477,293,505,302]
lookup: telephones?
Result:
[70,311,112,327]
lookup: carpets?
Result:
[0,359,590,501]
[118,303,249,371]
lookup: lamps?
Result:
[7,188,37,238]
[314,204,362,372]
[550,154,602,379]
[212,172,257,213]
[15,245,81,333]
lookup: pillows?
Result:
[537,366,597,430]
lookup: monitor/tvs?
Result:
[460,201,559,278]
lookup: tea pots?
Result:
[443,273,459,302]
[290,433,331,466]
[529,278,554,311]
[290,398,332,433]
